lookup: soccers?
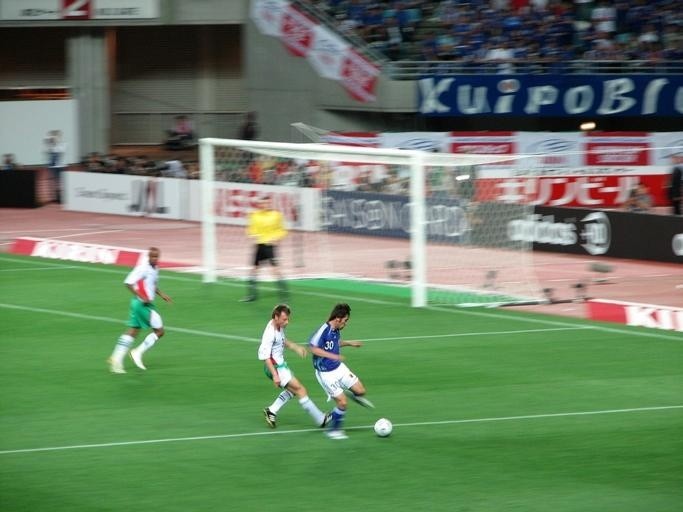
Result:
[373,418,393,436]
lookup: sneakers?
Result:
[129,348,147,370]
[263,407,277,428]
[107,355,128,374]
[323,430,349,440]
[319,412,335,429]
[352,392,374,408]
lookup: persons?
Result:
[228,149,475,200]
[257,304,327,428]
[81,152,194,178]
[1,153,16,165]
[240,112,260,165]
[293,0,683,71]
[166,115,193,150]
[624,167,683,217]
[310,303,375,439]
[108,246,171,373]
[238,198,288,303]
[41,128,65,166]
